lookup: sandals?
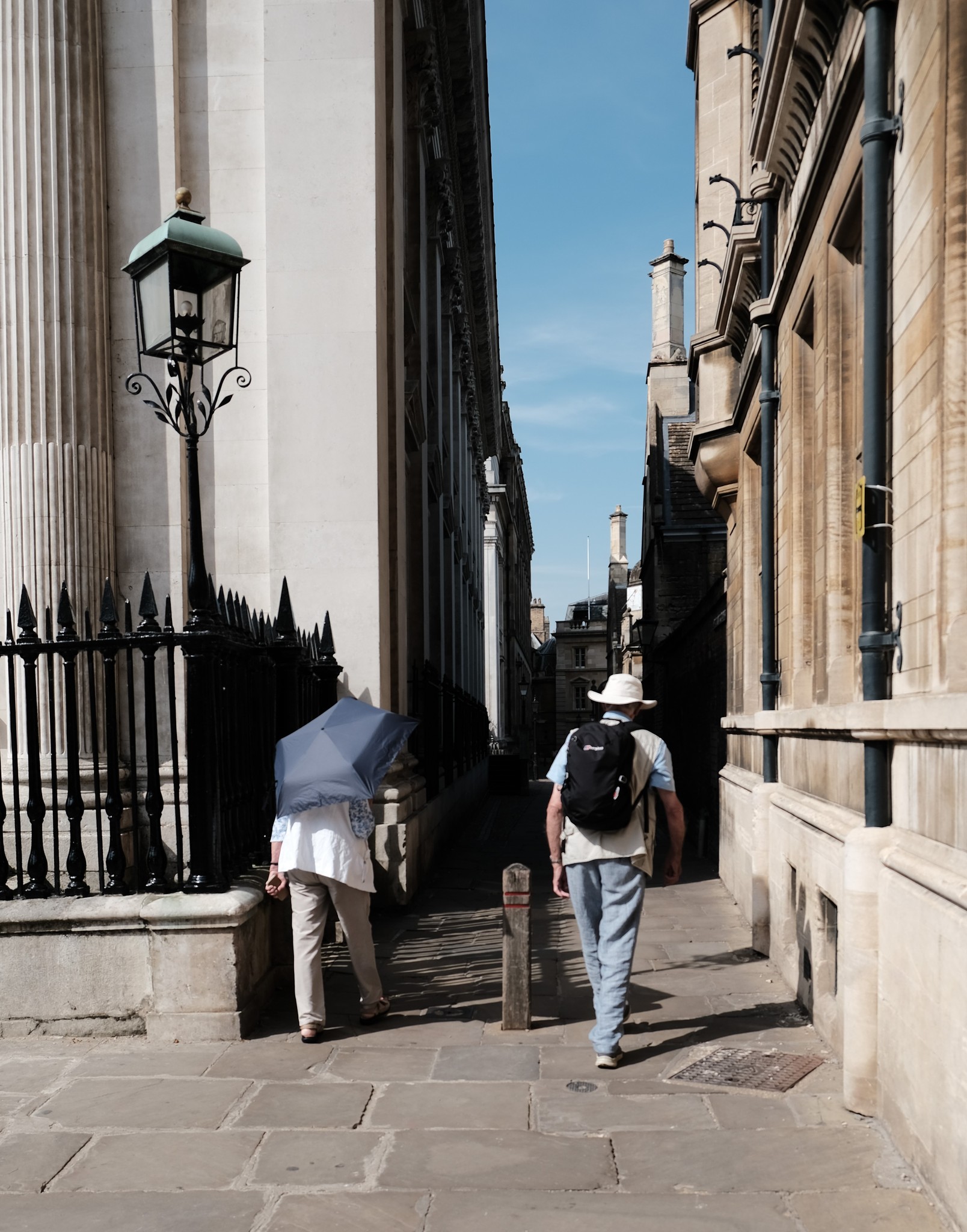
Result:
[300,1024,324,1043]
[359,997,390,1025]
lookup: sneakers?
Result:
[622,1000,630,1022]
[594,1046,623,1068]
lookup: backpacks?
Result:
[559,718,654,834]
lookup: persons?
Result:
[266,799,394,1044]
[546,674,685,1065]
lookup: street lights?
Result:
[118,185,251,897]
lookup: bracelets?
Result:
[270,861,279,866]
[550,856,561,864]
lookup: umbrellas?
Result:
[275,696,423,819]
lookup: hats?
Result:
[587,673,657,709]
